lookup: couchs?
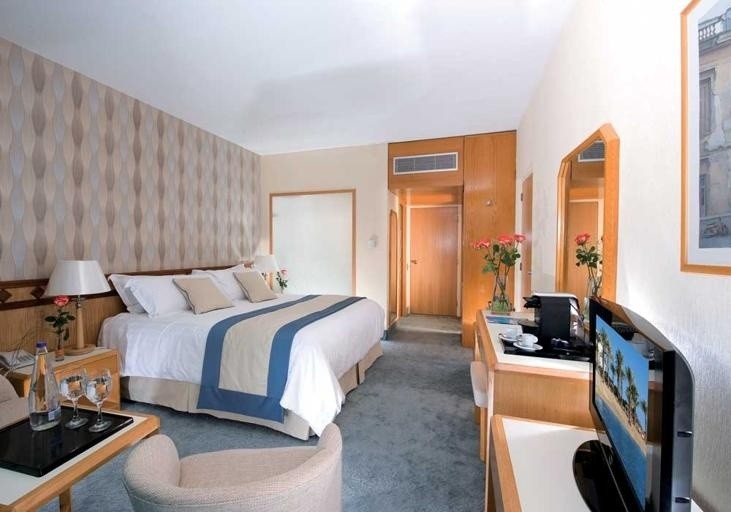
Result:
[0,375,33,427]
[123,422,342,512]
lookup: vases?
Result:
[54,331,66,361]
[582,277,607,318]
[280,288,285,294]
[491,270,510,315]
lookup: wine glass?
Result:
[58,367,87,430]
[80,368,112,433]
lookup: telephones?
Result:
[0,349,35,377]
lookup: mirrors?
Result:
[554,123,618,305]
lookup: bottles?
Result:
[28,341,62,431]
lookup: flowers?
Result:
[45,296,76,341]
[276,269,289,288]
[572,232,605,296]
[471,233,526,310]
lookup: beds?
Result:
[96,266,385,442]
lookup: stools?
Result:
[470,360,486,460]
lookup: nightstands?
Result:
[7,344,122,405]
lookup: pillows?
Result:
[107,263,278,314]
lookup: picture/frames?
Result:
[680,0,731,275]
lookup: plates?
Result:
[513,342,543,350]
[497,333,517,342]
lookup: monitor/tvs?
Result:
[571,294,697,512]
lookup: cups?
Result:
[516,334,533,348]
[502,326,519,339]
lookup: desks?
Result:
[475,305,599,460]
[491,416,703,512]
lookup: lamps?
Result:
[254,254,277,283]
[43,259,111,357]
